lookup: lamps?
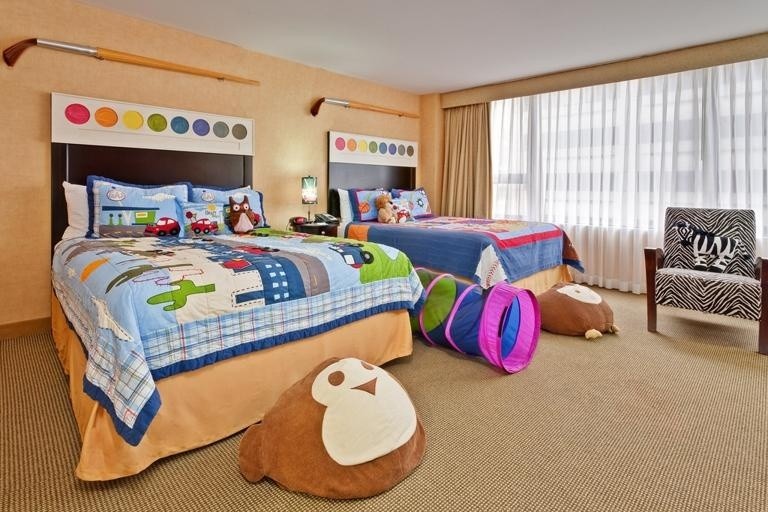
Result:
[301,175,318,223]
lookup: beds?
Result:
[52,91,426,481]
[328,130,585,296]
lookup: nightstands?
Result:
[291,222,340,237]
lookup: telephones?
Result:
[315,213,339,224]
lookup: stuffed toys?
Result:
[229,195,254,233]
[676,220,748,272]
[375,195,397,224]
[239,357,427,500]
[535,282,619,339]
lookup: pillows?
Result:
[337,187,433,224]
[61,174,271,240]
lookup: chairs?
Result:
[644,207,768,355]
